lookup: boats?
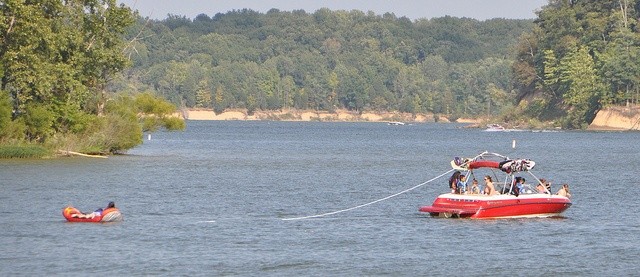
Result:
[417,149,573,219]
[61,201,122,223]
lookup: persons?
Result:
[555,182,569,197]
[69,199,117,218]
[484,173,496,195]
[469,176,481,193]
[456,174,466,194]
[510,176,533,194]
[535,178,548,193]
[448,170,461,193]
